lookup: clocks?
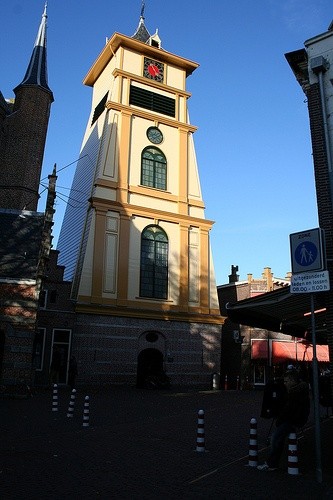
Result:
[143,57,164,84]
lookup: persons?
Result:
[258,360,333,472]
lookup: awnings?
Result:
[225,284,333,344]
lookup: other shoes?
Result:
[257,462,276,472]
